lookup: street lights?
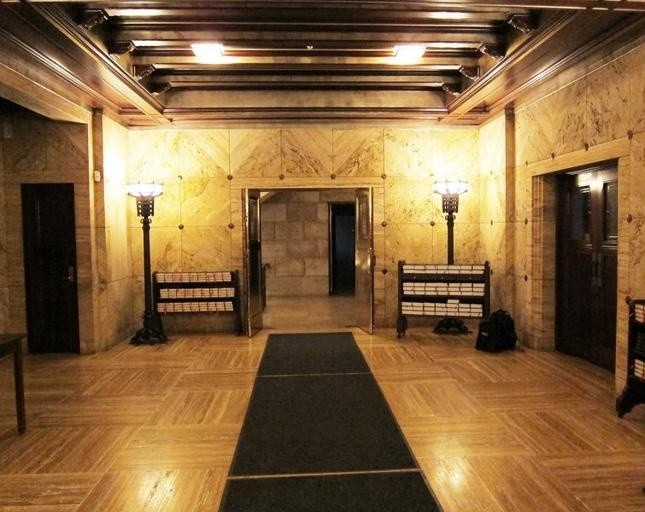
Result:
[125,181,163,345]
[431,180,469,264]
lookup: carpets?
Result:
[226,374,423,478]
[256,330,371,378]
[219,469,442,511]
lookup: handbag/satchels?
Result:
[475,309,516,353]
